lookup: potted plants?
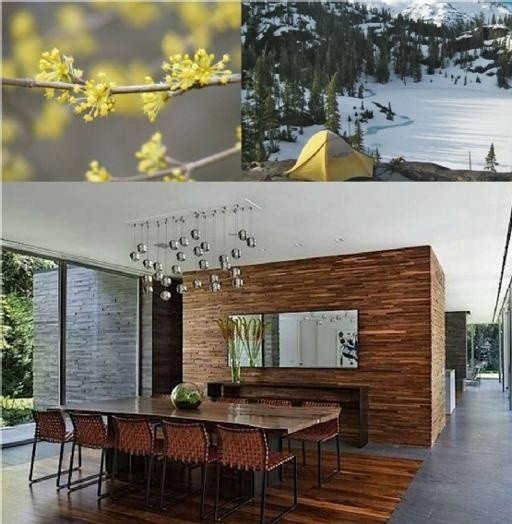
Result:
[212,314,242,383]
[235,316,273,367]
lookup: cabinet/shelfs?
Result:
[207,380,370,449]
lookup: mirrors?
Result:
[226,308,360,370]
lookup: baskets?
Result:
[171,382,202,409]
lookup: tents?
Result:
[283,130,376,182]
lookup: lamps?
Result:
[123,198,263,302]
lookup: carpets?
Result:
[0,446,423,523]
